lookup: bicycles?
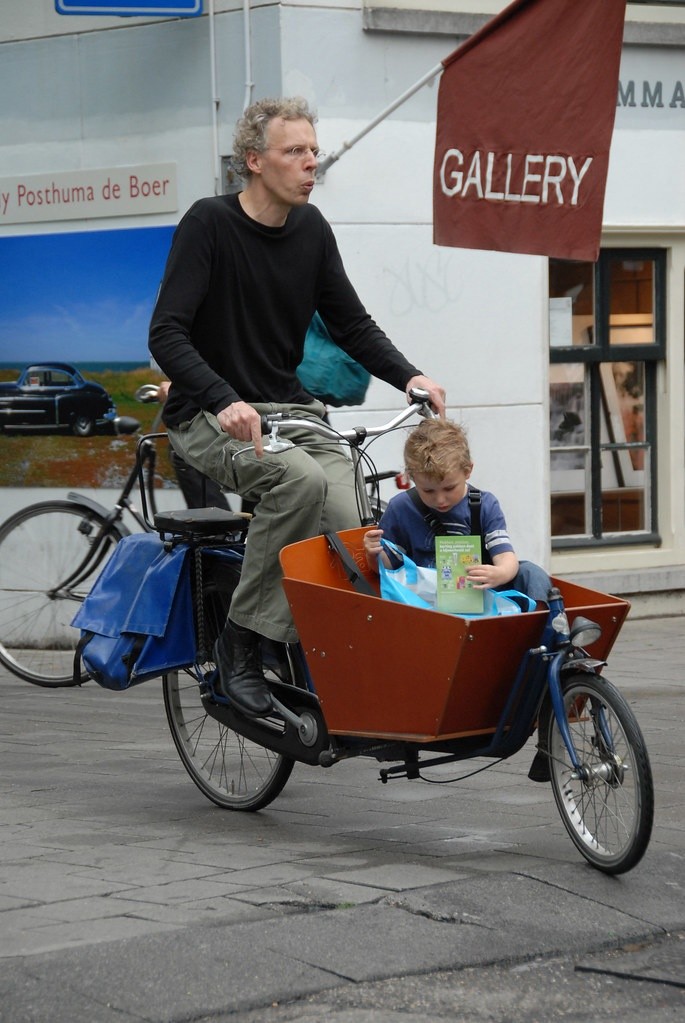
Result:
[0,382,412,689]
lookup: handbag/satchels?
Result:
[70,532,242,691]
[377,538,537,619]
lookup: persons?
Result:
[362,417,554,611]
[147,96,447,718]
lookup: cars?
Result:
[1,360,119,438]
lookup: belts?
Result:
[213,616,273,717]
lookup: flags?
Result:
[432,1,627,263]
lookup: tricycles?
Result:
[160,388,656,874]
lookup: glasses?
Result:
[262,146,325,162]
